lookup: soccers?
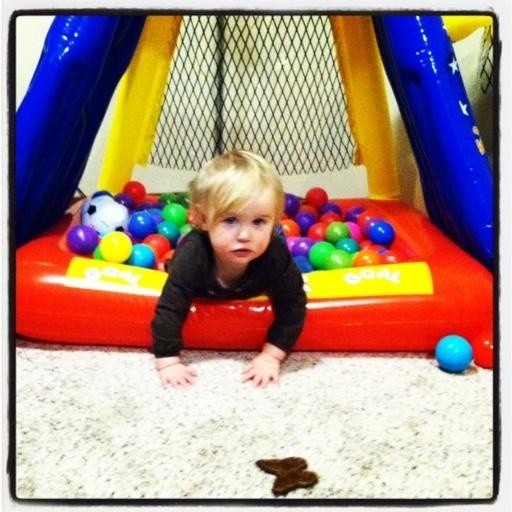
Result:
[81,195,129,238]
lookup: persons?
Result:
[150,151,307,390]
